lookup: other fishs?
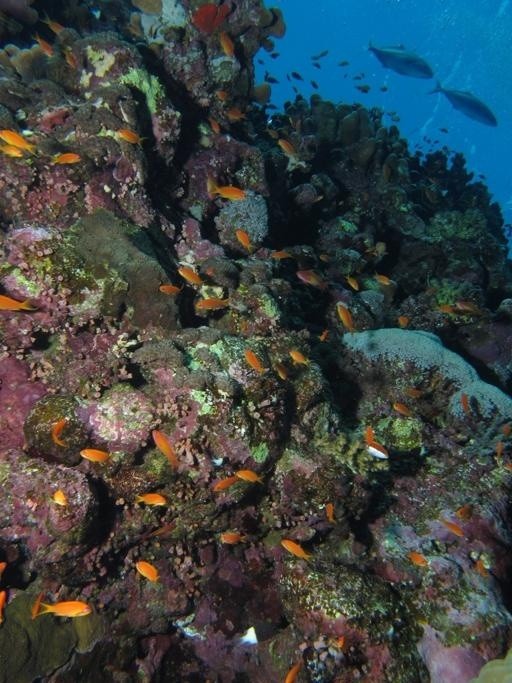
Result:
[1,2,512,683]
